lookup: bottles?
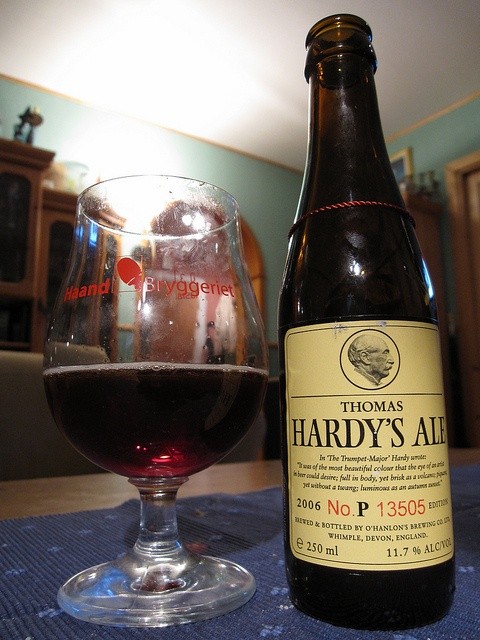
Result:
[278,14,455,631]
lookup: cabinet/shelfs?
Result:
[401,193,454,448]
[445,151,480,448]
[37,188,127,362]
[0,137,56,353]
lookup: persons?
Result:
[348,335,395,386]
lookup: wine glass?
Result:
[44,175,270,628]
